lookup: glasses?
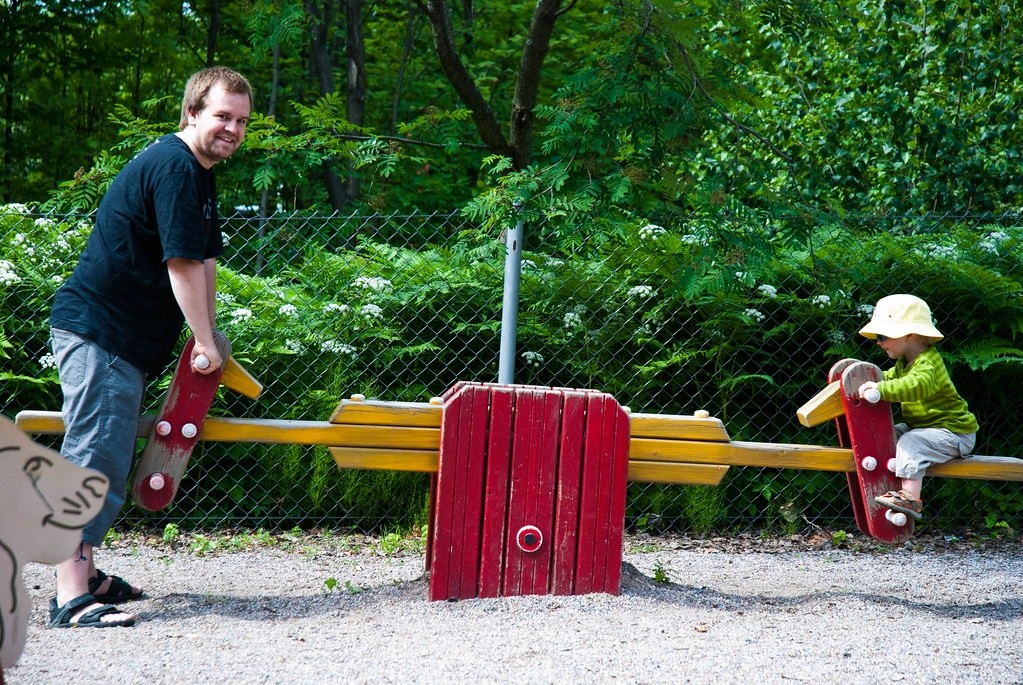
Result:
[876,333,888,341]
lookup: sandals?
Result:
[54,565,144,603]
[45,592,134,628]
[875,489,924,520]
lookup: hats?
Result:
[858,294,945,343]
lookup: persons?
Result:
[46,66,253,628]
[858,293,979,521]
